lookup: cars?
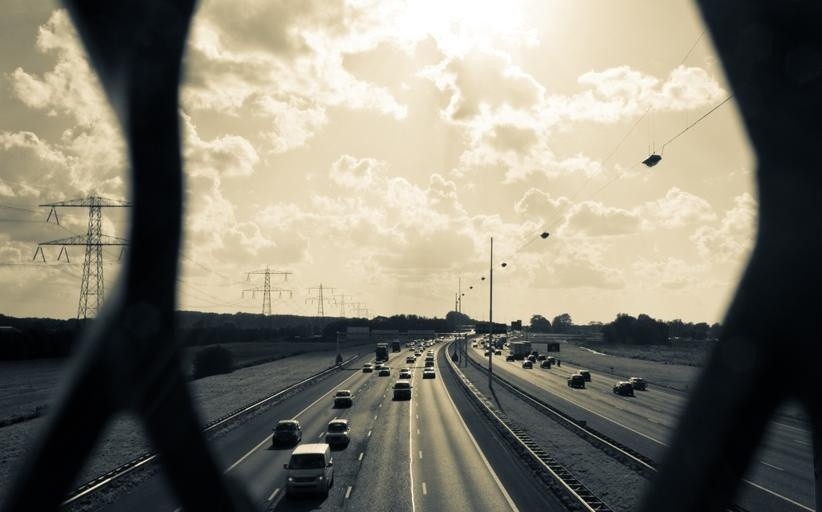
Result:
[471,329,649,397]
[272,335,459,500]
